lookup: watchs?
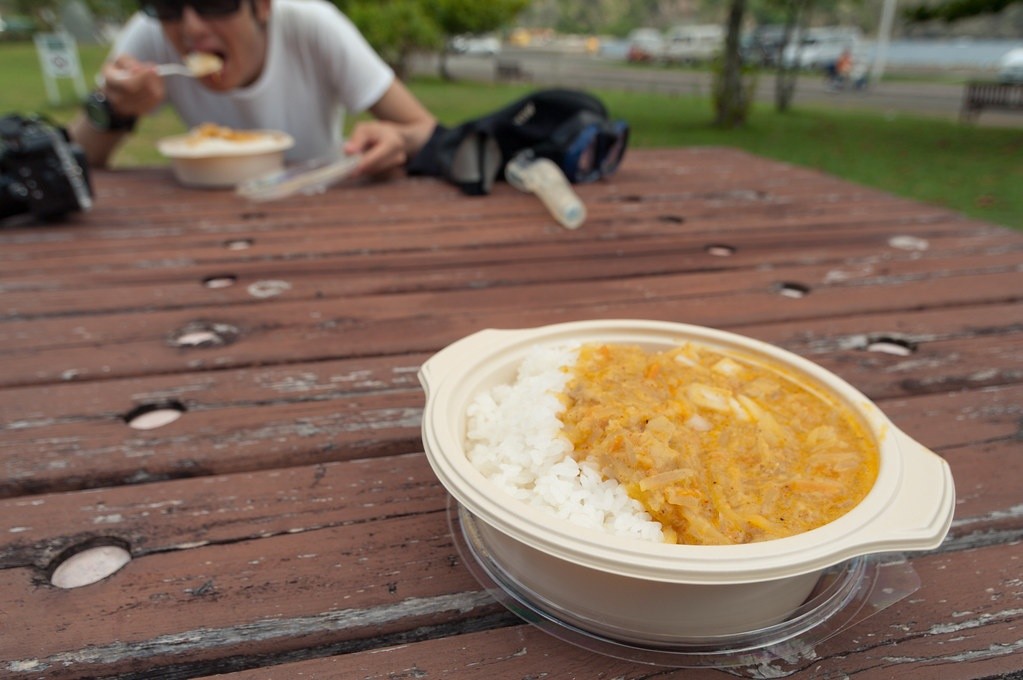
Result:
[83,95,136,132]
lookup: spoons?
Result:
[113,60,223,80]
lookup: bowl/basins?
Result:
[155,132,296,189]
[420,322,955,640]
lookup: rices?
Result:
[466,342,663,544]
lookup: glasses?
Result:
[139,0,241,21]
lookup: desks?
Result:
[0,143,1023,680]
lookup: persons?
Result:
[68,0,438,179]
